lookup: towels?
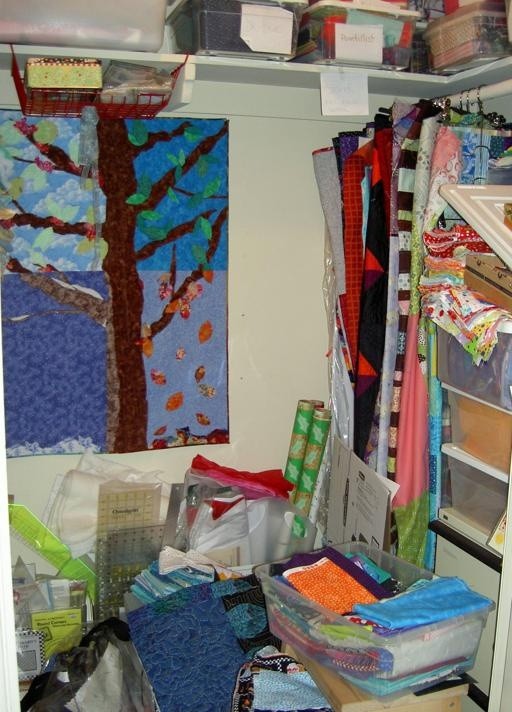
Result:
[310,574,493,697]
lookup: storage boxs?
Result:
[438,381,512,478]
[251,541,496,701]
[297,0,421,71]
[420,0,512,73]
[165,0,310,62]
[439,442,509,535]
[434,306,511,414]
[0,0,164,54]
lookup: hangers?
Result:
[431,84,505,132]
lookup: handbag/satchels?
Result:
[23,618,154,712]
